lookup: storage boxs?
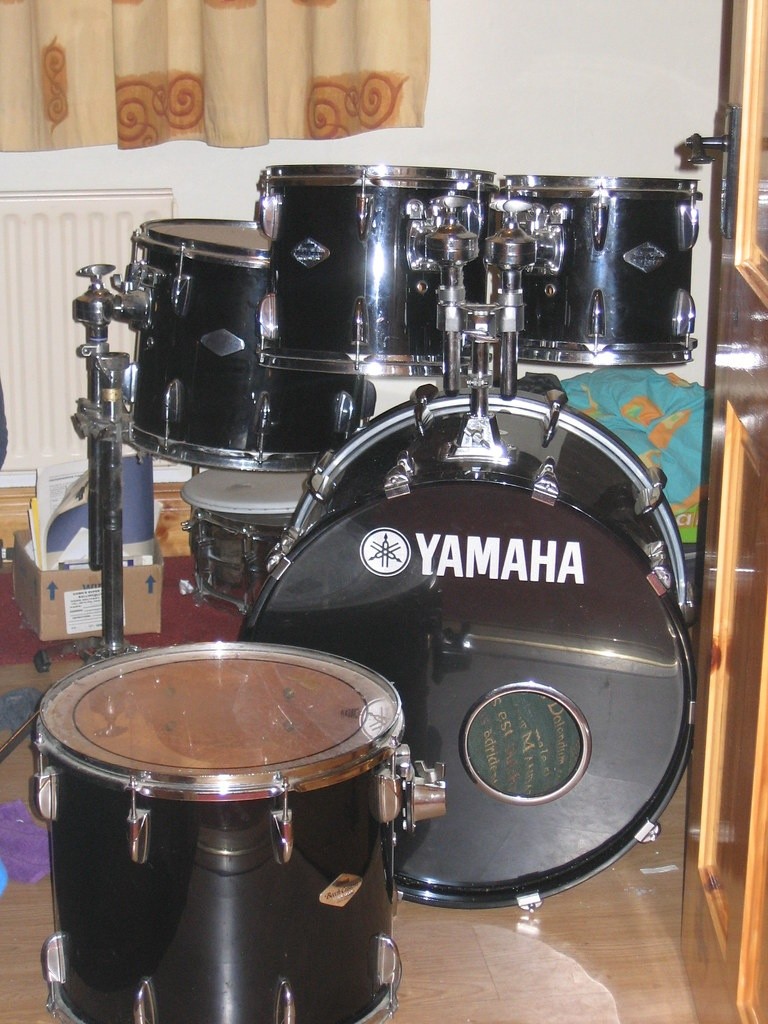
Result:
[12,528,166,642]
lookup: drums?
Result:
[493,176,700,368]
[28,641,407,1024]
[240,385,696,912]
[181,468,316,612]
[257,162,501,373]
[123,219,368,469]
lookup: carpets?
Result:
[0,556,246,665]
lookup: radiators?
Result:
[0,188,180,471]
